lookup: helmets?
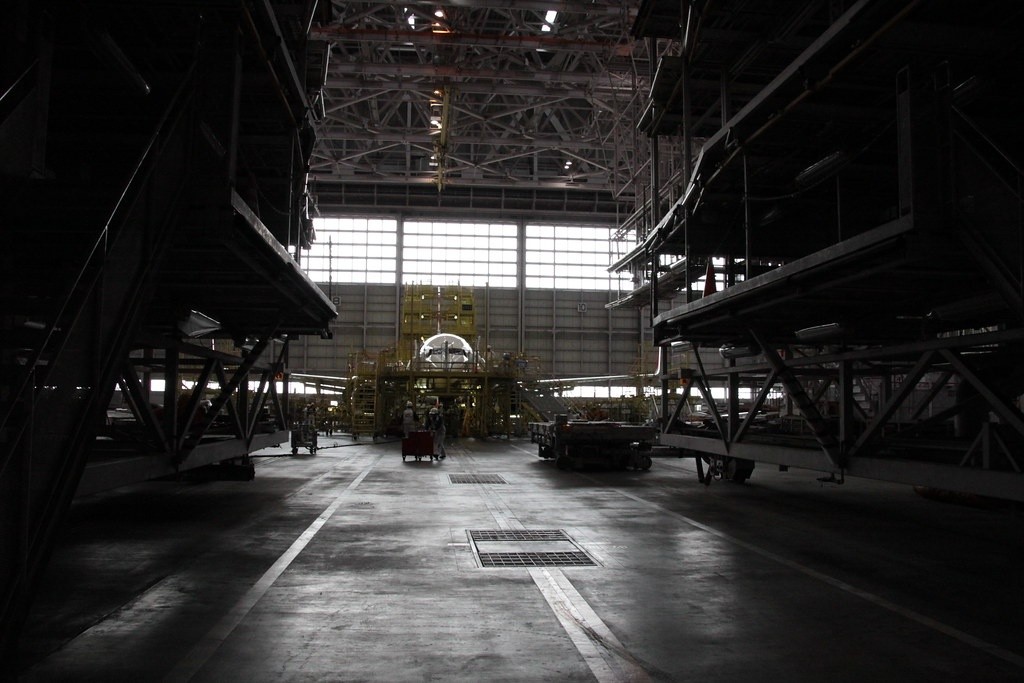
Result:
[429,408,438,414]
[406,402,413,406]
[307,404,310,406]
[311,403,314,405]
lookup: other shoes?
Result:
[439,456,446,458]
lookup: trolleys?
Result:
[401,431,433,462]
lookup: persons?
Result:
[400,400,422,439]
[424,408,447,459]
[484,345,495,370]
